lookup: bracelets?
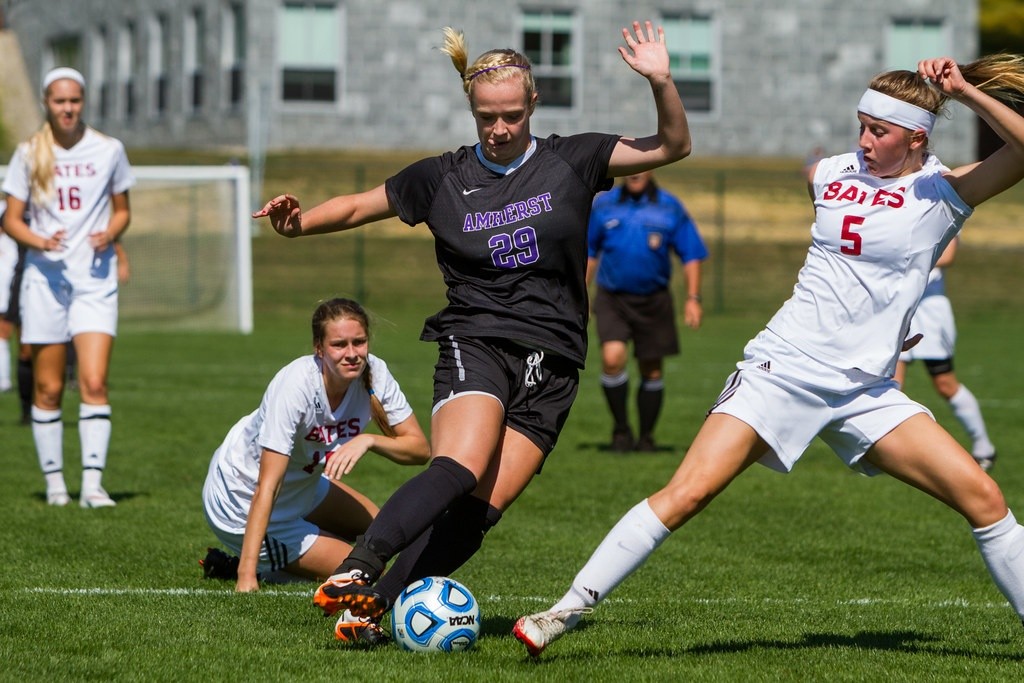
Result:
[685,292,703,302]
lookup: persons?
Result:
[2,165,129,425]
[891,229,1002,477]
[582,172,708,450]
[511,47,1024,653]
[200,295,429,596]
[250,19,688,647]
[4,67,134,509]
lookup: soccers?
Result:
[389,576,480,652]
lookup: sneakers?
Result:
[199,547,237,580]
[313,573,389,618]
[336,603,393,645]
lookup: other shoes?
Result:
[79,485,116,508]
[636,437,656,451]
[612,433,634,450]
[511,606,576,656]
[46,486,73,507]
[972,446,997,474]
